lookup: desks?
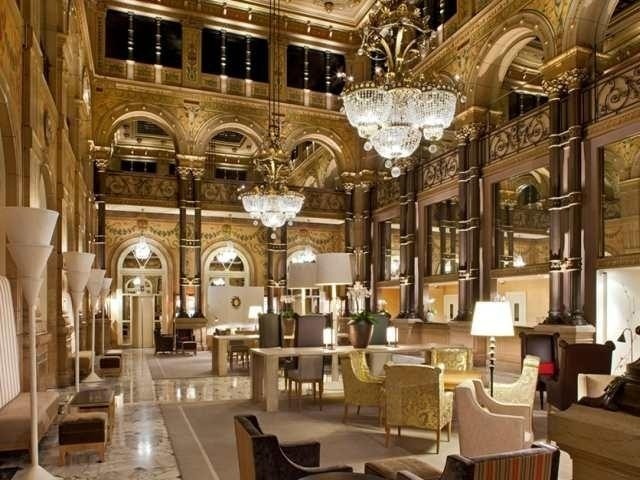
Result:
[68,389,115,441]
[104,347,122,367]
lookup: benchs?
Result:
[0,391,59,451]
[72,351,92,373]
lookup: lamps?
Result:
[314,254,355,382]
[289,264,313,316]
[339,0,458,178]
[470,301,515,399]
[291,218,316,264]
[213,213,235,265]
[236,0,306,242]
[131,209,151,261]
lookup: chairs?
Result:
[176,328,196,355]
[154,327,174,355]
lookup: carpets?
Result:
[146,351,250,380]
[161,389,551,480]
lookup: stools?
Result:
[60,412,108,467]
[100,356,122,377]
